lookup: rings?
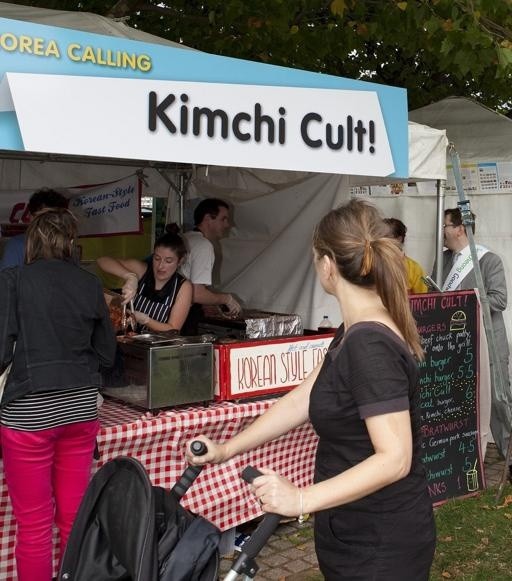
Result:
[258,495,268,505]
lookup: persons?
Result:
[183,203,441,581]
[171,196,244,336]
[1,208,119,581]
[2,184,76,268]
[375,216,428,295]
[94,222,196,335]
[430,208,512,480]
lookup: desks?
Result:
[0,395,319,581]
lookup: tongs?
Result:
[164,329,218,344]
[120,290,136,332]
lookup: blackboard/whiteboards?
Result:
[409,288,487,508]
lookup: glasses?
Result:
[444,224,460,228]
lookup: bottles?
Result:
[319,315,331,328]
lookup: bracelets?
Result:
[124,273,138,279]
[226,293,232,308]
[296,486,306,526]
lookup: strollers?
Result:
[57,439,283,581]
